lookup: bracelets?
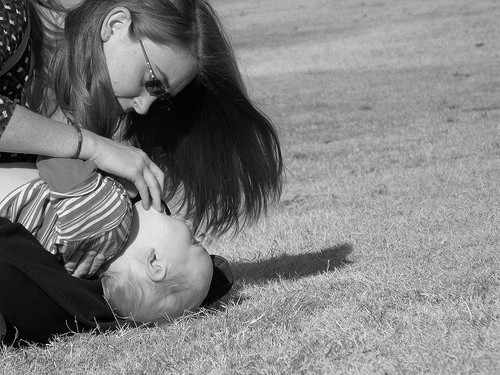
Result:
[70,121,83,159]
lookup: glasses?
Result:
[130,21,173,108]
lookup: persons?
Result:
[1,0,288,246]
[0,151,214,327]
